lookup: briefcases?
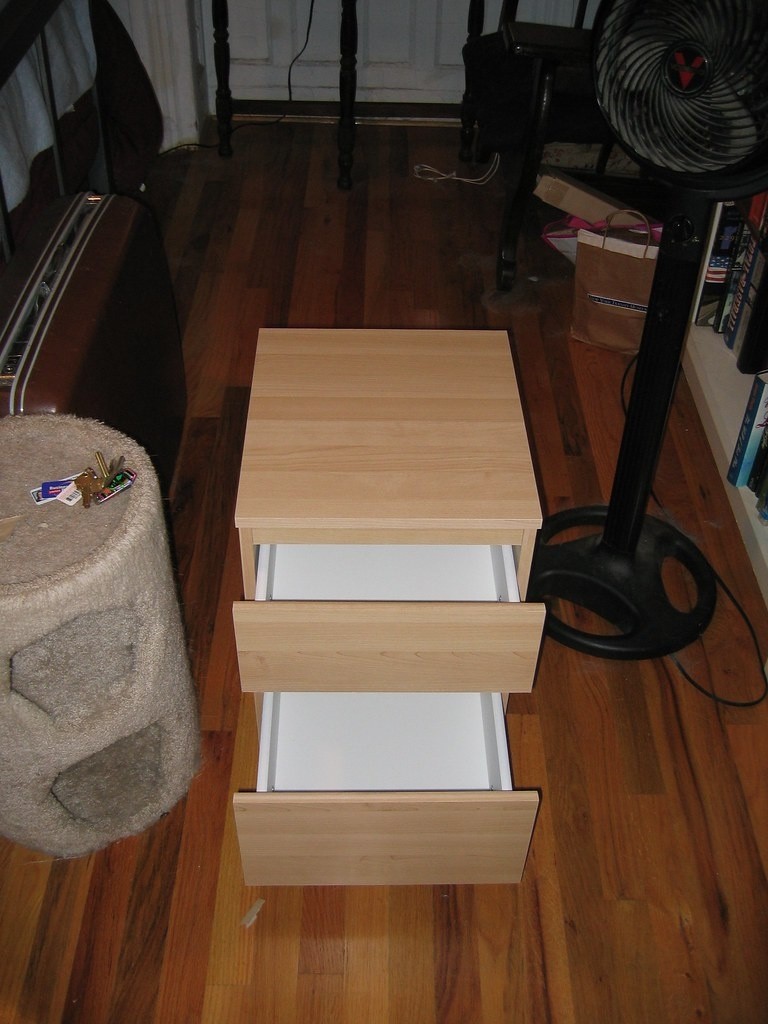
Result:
[0,190,187,513]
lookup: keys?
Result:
[74,452,137,509]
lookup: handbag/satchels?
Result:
[505,22,589,58]
[570,209,661,356]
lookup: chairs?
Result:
[496,0,618,292]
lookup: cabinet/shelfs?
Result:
[232,325,549,883]
[680,192,767,613]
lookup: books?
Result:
[726,370,768,526]
[712,220,768,374]
